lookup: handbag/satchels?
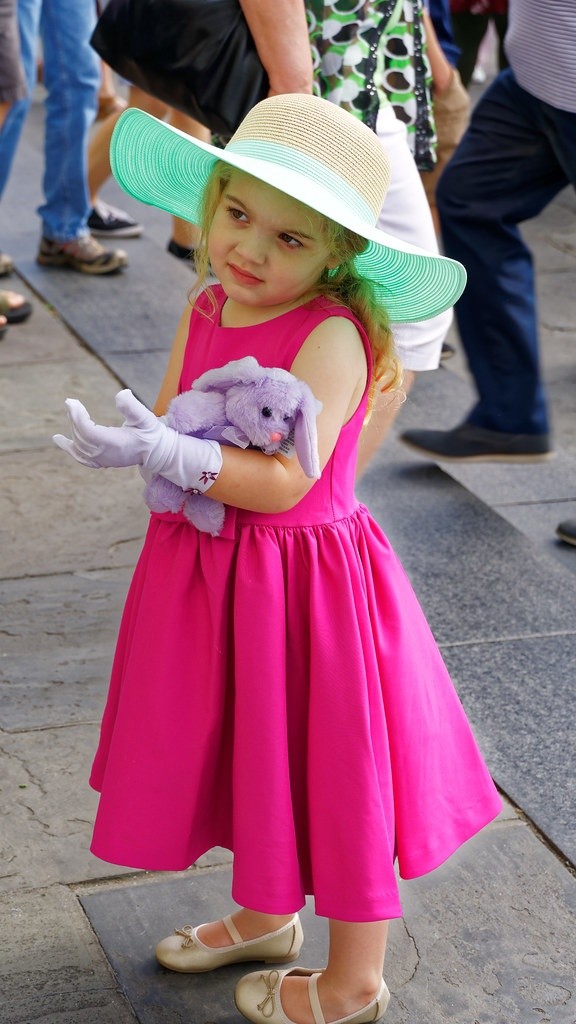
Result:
[90,2,276,137]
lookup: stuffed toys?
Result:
[142,356,323,538]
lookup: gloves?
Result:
[52,389,222,495]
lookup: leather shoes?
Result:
[395,397,557,458]
[554,516,576,547]
[233,966,390,1024]
[155,912,306,974]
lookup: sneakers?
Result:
[36,234,127,277]
[167,240,215,276]
[89,200,143,238]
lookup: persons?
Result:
[415,0,511,223]
[85,0,270,276]
[0,0,25,339]
[0,0,130,276]
[53,93,504,1024]
[237,1,455,482]
[397,0,576,550]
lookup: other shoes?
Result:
[1,252,13,277]
[0,288,32,335]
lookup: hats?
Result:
[108,92,467,324]
